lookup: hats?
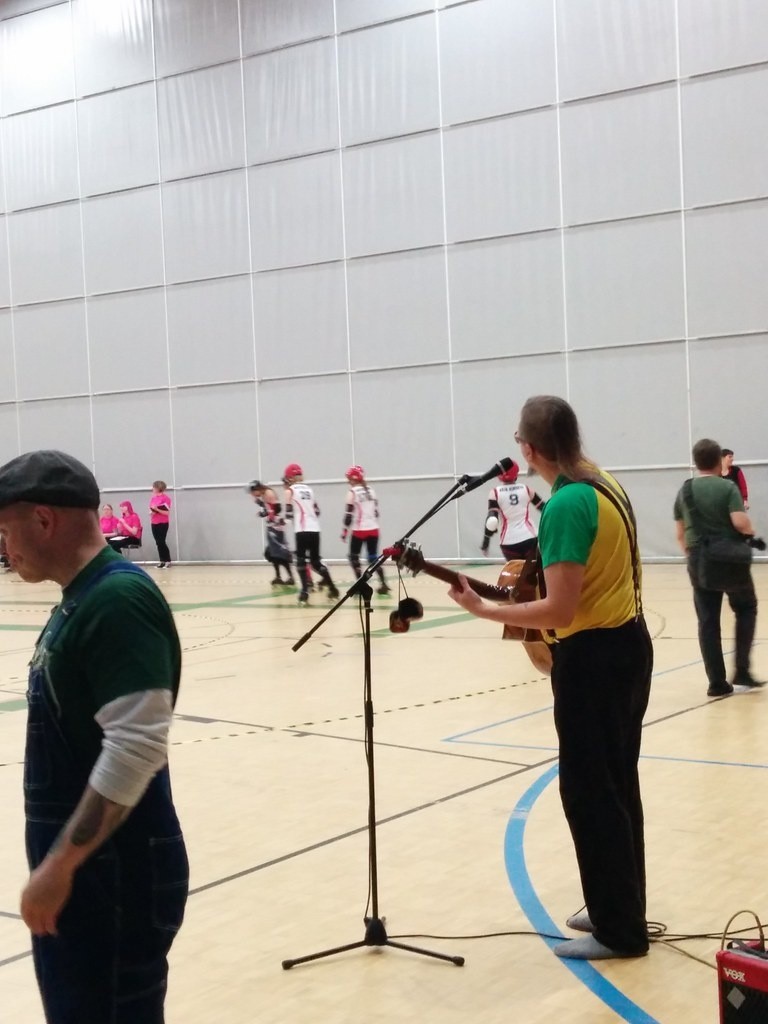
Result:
[0,451,101,509]
[247,481,261,493]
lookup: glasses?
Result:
[515,433,527,448]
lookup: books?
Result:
[150,505,169,514]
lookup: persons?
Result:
[247,480,294,588]
[100,501,141,554]
[482,461,544,561]
[282,464,341,607]
[0,449,189,1024]
[339,466,391,599]
[447,395,653,960]
[673,438,768,697]
[720,449,750,510]
[149,481,171,569]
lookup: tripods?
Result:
[280,474,482,970]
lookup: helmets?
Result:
[346,466,365,482]
[497,459,519,481]
[285,464,303,479]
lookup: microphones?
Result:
[452,457,514,499]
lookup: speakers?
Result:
[716,940,768,1024]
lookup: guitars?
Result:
[392,539,553,675]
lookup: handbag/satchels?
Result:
[697,538,753,593]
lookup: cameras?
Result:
[743,534,765,551]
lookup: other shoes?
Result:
[378,585,388,595]
[327,588,340,599]
[162,562,171,569]
[297,592,309,602]
[156,560,166,568]
[735,673,762,687]
[270,578,296,585]
[705,679,732,696]
